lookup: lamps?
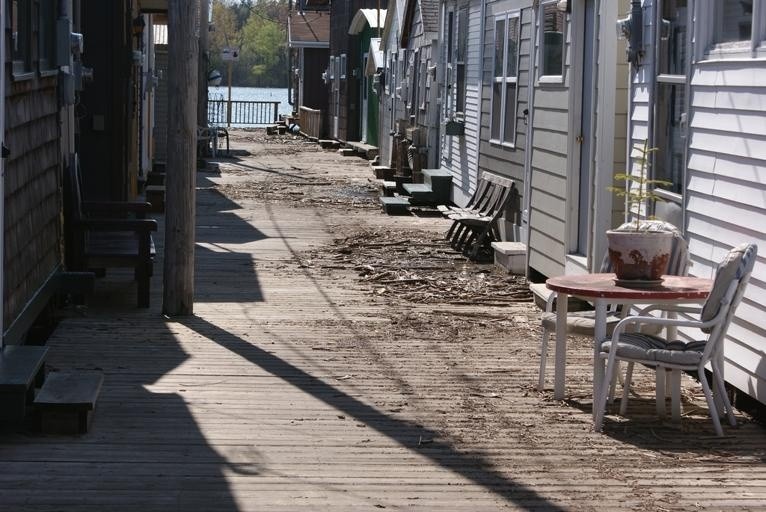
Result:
[132,15,145,38]
[207,68,221,86]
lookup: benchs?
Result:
[436,170,514,255]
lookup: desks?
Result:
[545,272,714,424]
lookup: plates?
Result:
[612,276,665,288]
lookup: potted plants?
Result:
[605,138,674,281]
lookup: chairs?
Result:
[67,151,156,308]
[196,125,217,158]
[593,242,757,438]
[528,220,690,406]
